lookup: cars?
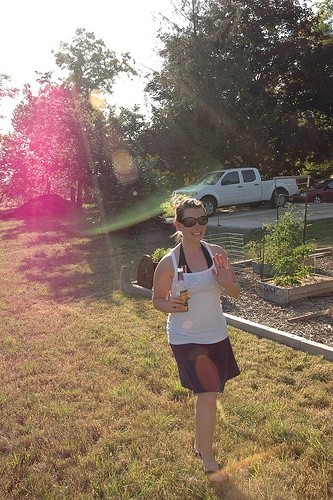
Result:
[298,180,333,203]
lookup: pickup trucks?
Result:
[170,168,298,217]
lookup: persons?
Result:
[152,193,242,483]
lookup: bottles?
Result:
[175,267,188,313]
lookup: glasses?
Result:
[176,216,208,227]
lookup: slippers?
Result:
[202,463,228,484]
[194,442,203,459]
